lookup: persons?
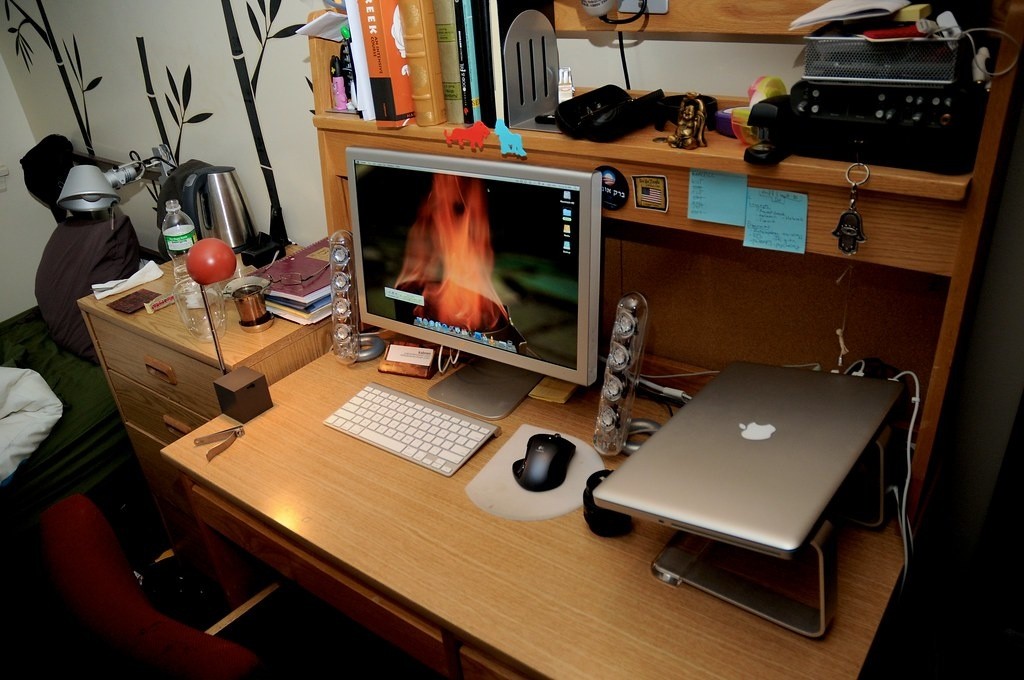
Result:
[393,175,527,352]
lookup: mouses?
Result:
[512,432,577,493]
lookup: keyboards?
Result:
[322,381,506,479]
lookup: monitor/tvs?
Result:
[346,147,602,420]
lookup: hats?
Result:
[19,134,74,224]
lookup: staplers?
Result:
[742,94,797,167]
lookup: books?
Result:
[257,239,332,326]
[357,0,559,129]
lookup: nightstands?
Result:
[78,242,339,593]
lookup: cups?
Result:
[222,285,274,333]
[172,277,226,343]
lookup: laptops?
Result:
[592,359,906,559]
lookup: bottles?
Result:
[162,199,197,283]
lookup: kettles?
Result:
[182,166,256,254]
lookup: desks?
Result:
[159,322,927,680]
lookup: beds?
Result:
[0,154,183,598]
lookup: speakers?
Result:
[596,293,663,457]
[330,229,385,365]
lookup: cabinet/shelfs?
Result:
[308,0,1024,521]
